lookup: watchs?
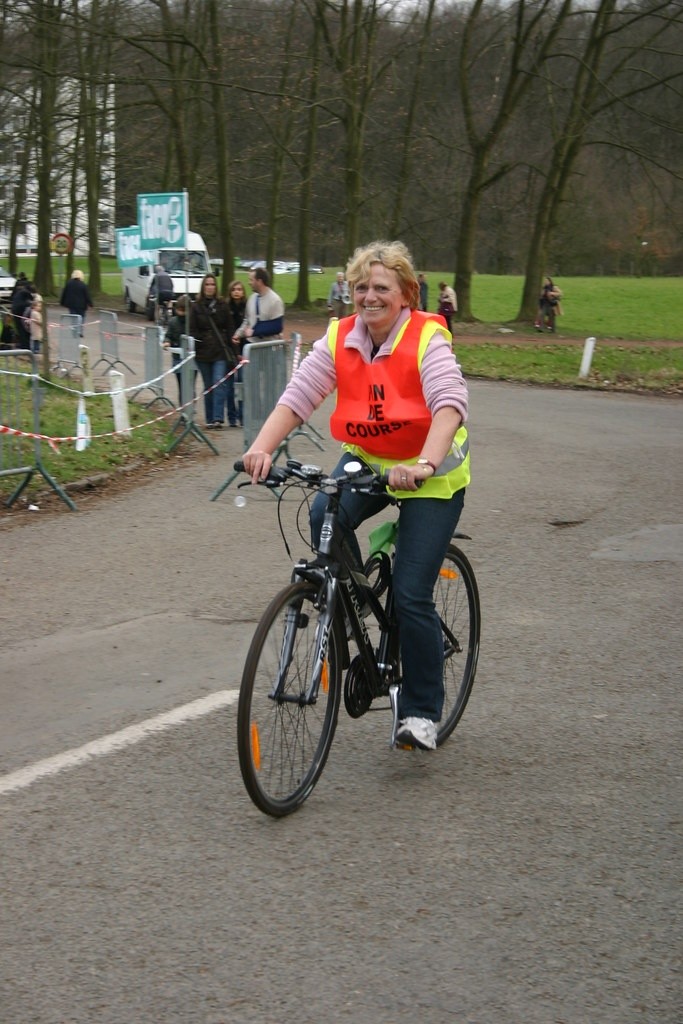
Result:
[417,459,435,473]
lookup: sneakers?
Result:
[315,571,372,644]
[394,716,437,751]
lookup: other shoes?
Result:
[238,418,244,427]
[214,421,222,429]
[207,423,214,429]
[229,418,236,426]
[548,326,552,329]
[535,325,540,327]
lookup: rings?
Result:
[402,477,406,479]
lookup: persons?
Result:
[418,275,427,310]
[60,270,93,337]
[326,272,352,318]
[189,274,230,429]
[231,269,284,352]
[241,240,472,751]
[148,267,172,301]
[11,272,43,353]
[225,280,248,427]
[438,282,457,336]
[534,278,561,330]
[161,295,197,413]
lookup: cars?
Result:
[209,257,325,276]
[0,265,18,303]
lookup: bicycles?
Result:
[235,454,483,821]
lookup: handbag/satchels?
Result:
[437,302,454,316]
[225,346,236,363]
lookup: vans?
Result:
[118,223,220,322]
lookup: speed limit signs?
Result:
[51,232,74,254]
